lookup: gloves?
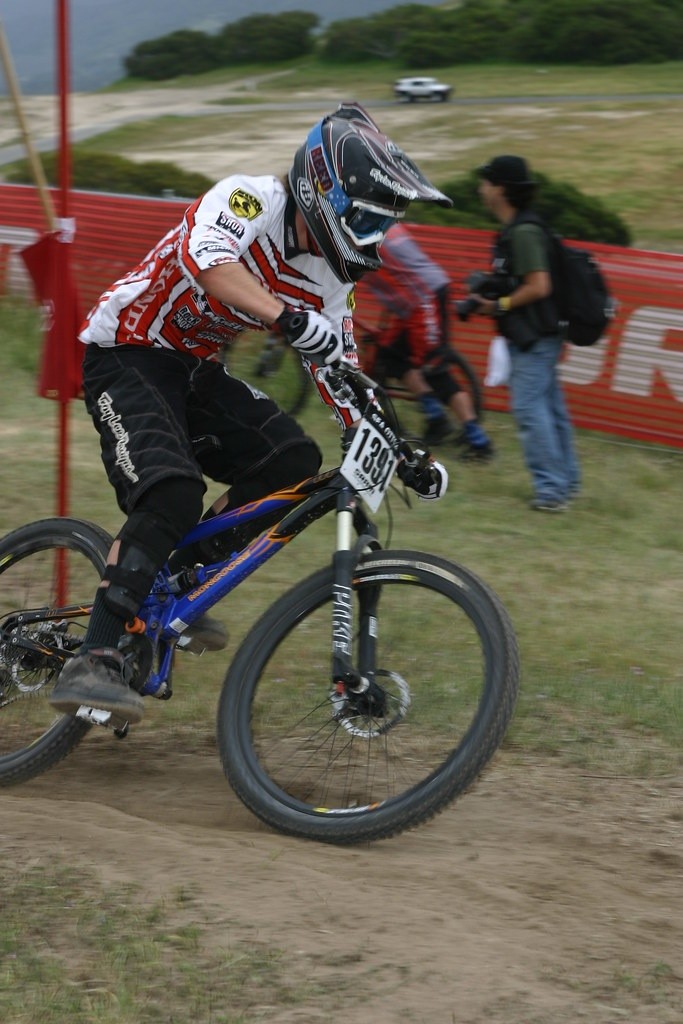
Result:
[396,455,449,502]
[271,306,342,366]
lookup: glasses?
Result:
[340,197,406,247]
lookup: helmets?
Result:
[290,101,451,284]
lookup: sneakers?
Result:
[529,496,569,512]
[179,616,230,653]
[460,443,496,464]
[49,647,147,727]
[419,416,460,445]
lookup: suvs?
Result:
[395,77,451,103]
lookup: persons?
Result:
[50,100,454,726]
[470,155,580,512]
[364,223,493,459]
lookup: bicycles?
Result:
[223,316,480,431]
[0,312,521,847]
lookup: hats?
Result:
[474,155,545,194]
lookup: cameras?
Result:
[448,254,521,323]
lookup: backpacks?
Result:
[508,219,614,348]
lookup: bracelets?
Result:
[496,296,510,316]
